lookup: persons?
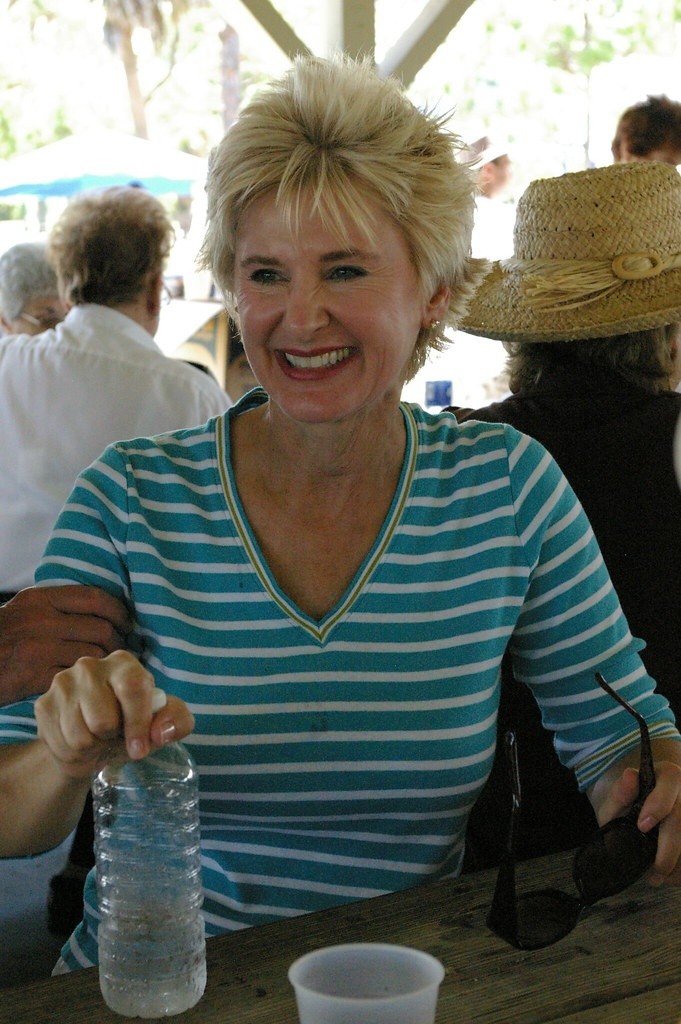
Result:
[0,95,681,871]
[0,62,681,974]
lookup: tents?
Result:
[0,129,210,234]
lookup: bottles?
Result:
[93,686,208,1019]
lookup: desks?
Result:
[0,846,681,1024]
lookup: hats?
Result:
[449,159,681,343]
[466,137,507,170]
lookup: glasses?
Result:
[19,312,63,332]
[160,280,173,306]
[485,674,658,952]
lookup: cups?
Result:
[289,943,444,1024]
